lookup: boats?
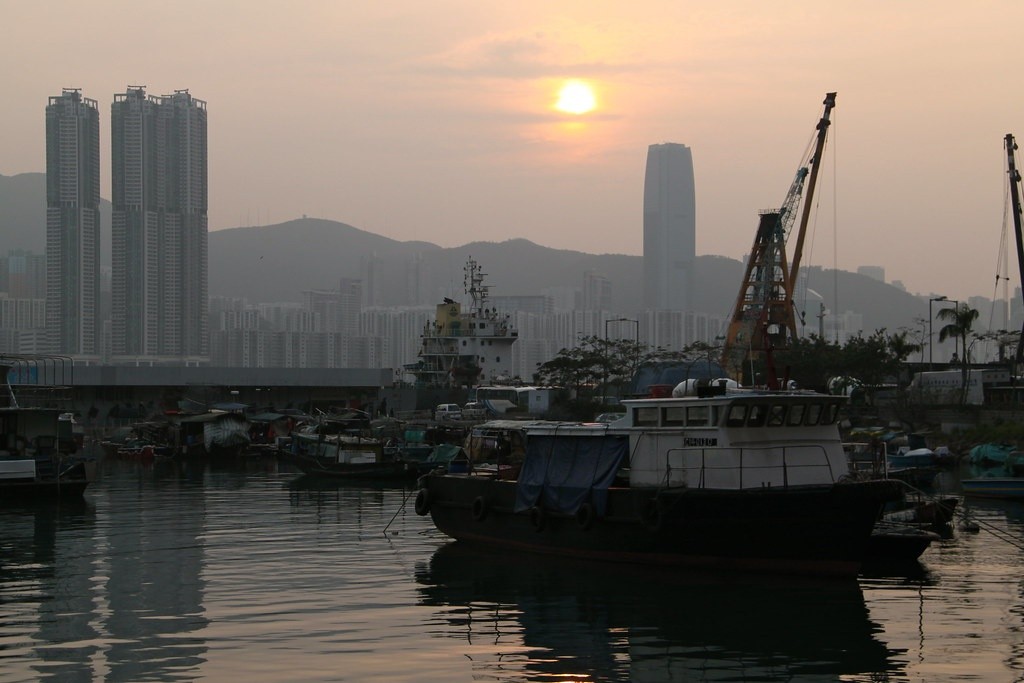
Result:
[0,254,965,595]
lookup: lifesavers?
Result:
[415,488,432,516]
[640,498,664,535]
[527,507,546,533]
[576,505,594,531]
[471,495,489,523]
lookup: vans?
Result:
[461,402,486,420]
[433,404,461,420]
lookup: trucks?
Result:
[906,369,1010,407]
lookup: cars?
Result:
[592,397,620,407]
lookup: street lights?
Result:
[930,295,947,372]
[603,318,639,406]
[935,300,958,362]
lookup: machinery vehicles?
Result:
[642,378,743,400]
[779,375,866,403]
